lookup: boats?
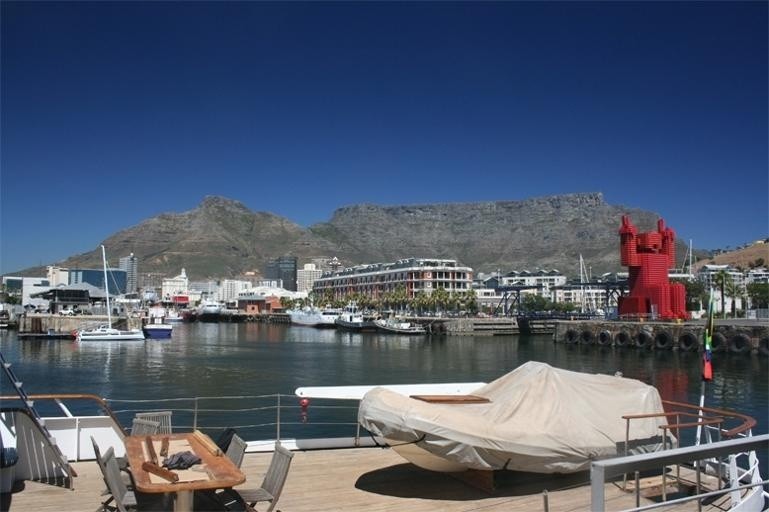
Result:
[143,316,173,339]
[17,329,76,339]
[313,300,428,334]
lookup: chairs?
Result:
[90,409,295,512]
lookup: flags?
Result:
[702,292,713,381]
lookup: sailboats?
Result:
[75,244,145,341]
